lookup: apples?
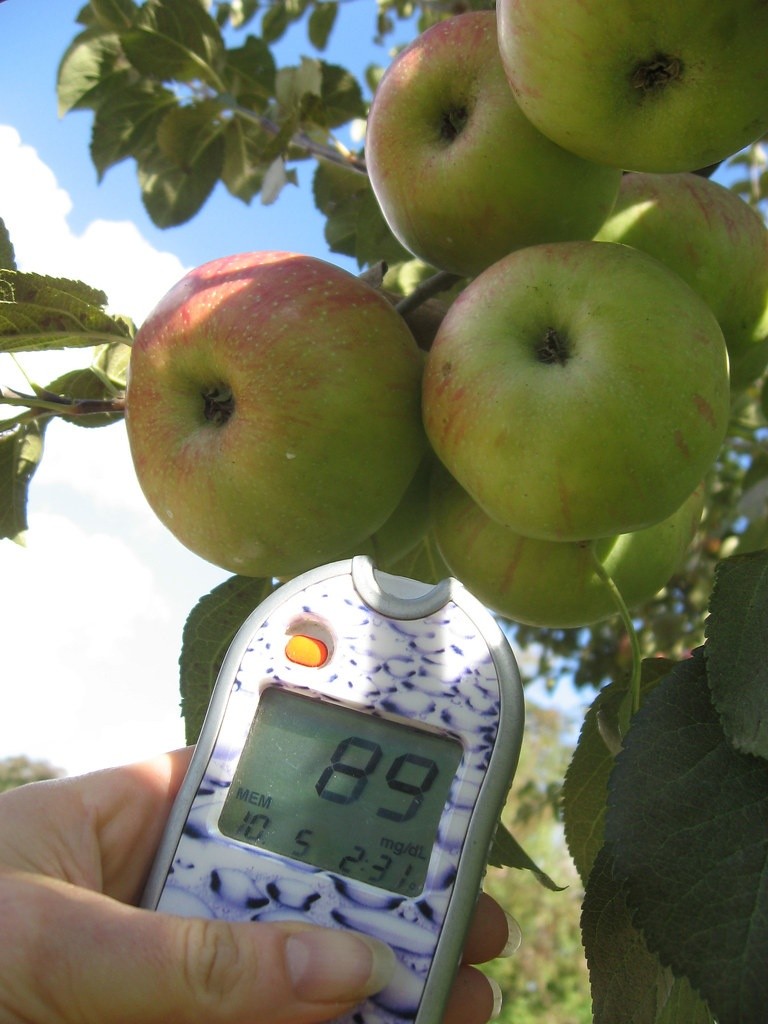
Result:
[121,0,768,629]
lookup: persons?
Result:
[0,743,522,1024]
